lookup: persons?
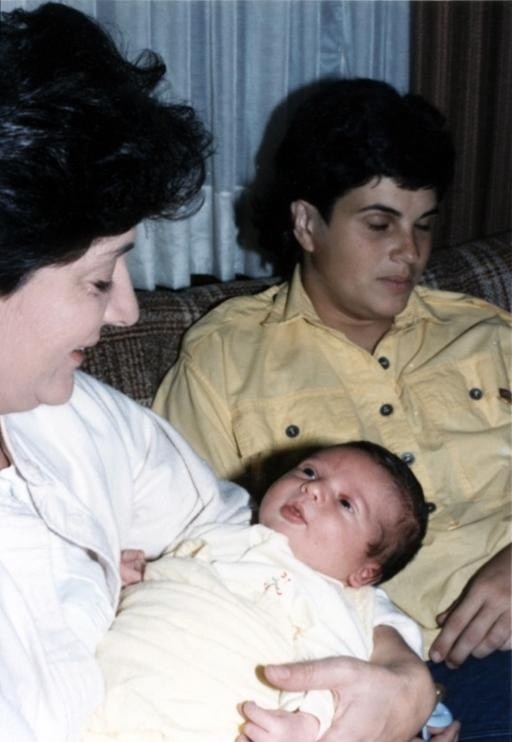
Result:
[0,1,439,741]
[94,436,429,740]
[149,75,512,741]
